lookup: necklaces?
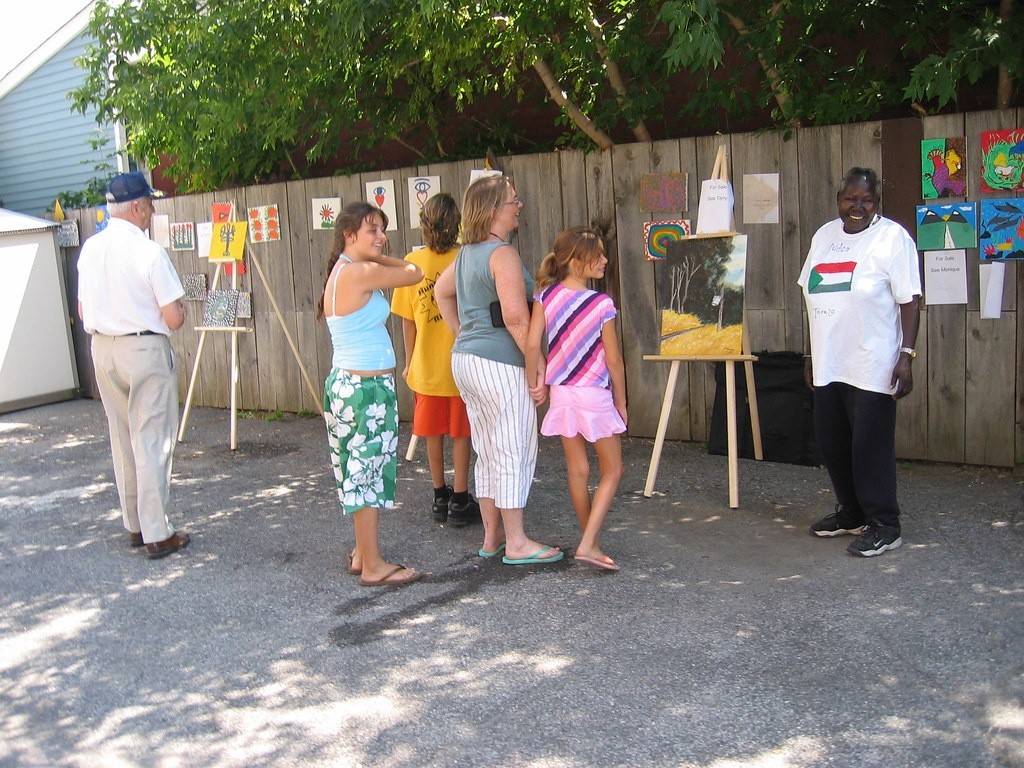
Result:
[490,232,504,242]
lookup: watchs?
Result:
[900,347,917,358]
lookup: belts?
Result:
[131,330,154,336]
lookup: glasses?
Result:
[495,195,520,209]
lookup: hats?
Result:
[104,172,158,203]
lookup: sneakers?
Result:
[447,495,483,528]
[432,485,457,522]
[810,502,868,538]
[846,522,903,558]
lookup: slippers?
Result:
[359,560,423,585]
[478,543,508,558]
[502,543,565,564]
[348,549,364,576]
[574,549,623,571]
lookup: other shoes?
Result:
[130,531,146,548]
[147,531,191,558]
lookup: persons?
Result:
[797,167,923,558]
[76,171,190,559]
[390,193,481,526]
[316,202,425,586]
[525,225,628,569]
[435,174,564,564]
